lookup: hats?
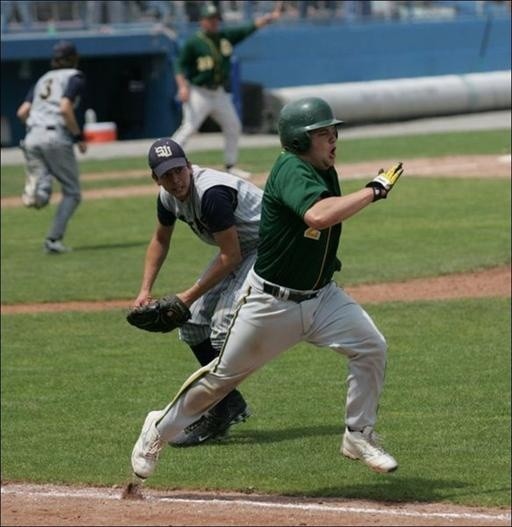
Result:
[197,5,221,18]
[147,137,188,178]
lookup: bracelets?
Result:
[71,132,84,144]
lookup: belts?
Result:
[263,280,325,304]
[45,125,70,131]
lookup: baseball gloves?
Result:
[128,291,192,334]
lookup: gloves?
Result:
[366,162,408,201]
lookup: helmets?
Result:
[278,95,348,155]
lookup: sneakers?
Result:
[228,166,253,181]
[339,425,399,473]
[131,409,169,480]
[43,237,74,254]
[214,390,250,424]
[165,417,230,448]
[21,175,39,207]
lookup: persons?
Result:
[168,1,282,180]
[123,135,268,448]
[128,97,408,480]
[14,37,91,254]
